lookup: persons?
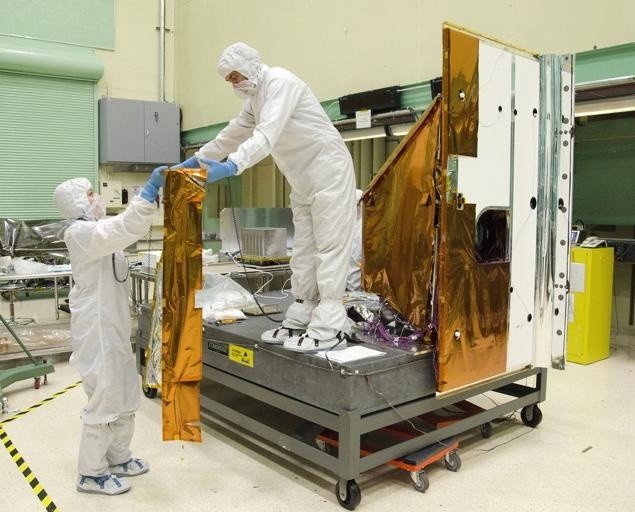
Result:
[160,41,361,355]
[50,164,171,497]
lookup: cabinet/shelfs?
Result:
[98,96,181,166]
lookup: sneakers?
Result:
[109,457,149,476]
[261,325,306,344]
[76,474,131,495]
[282,329,348,354]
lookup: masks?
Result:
[86,193,106,220]
[232,78,260,99]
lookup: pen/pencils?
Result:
[215,320,242,324]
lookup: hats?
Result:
[216,42,263,99]
[52,177,95,220]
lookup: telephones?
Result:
[580,236,607,248]
